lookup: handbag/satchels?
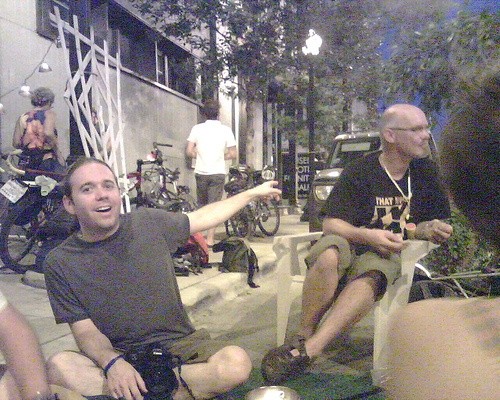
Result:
[212,237,258,273]
[184,233,210,265]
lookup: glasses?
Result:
[390,124,431,138]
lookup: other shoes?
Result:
[140,366,176,400]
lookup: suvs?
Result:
[307,129,441,246]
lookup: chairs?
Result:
[271,231,441,386]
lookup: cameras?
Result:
[132,348,179,400]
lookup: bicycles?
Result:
[0,147,80,272]
[223,164,281,238]
[120,141,210,271]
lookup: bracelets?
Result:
[103,355,123,375]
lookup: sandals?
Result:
[261,336,310,384]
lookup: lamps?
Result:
[19,84,33,96]
[39,61,53,73]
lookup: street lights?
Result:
[301,28,324,222]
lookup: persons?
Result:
[44,157,284,400]
[13,88,66,245]
[187,100,238,247]
[260,104,454,383]
[0,291,54,400]
[90,111,113,159]
[373,67,500,400]
